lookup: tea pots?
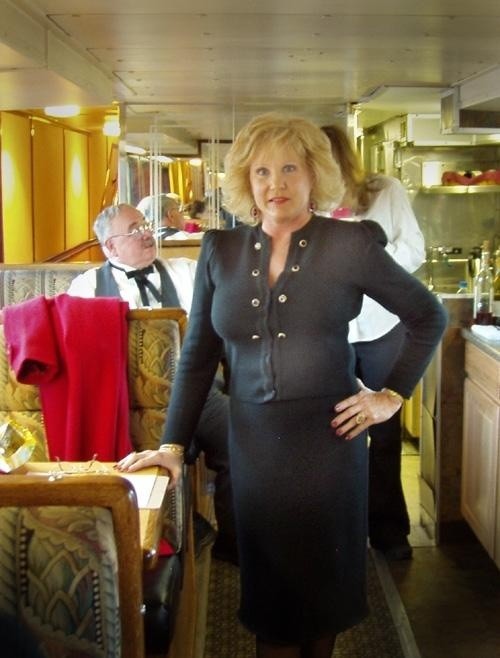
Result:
[467,247,481,292]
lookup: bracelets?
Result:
[381,388,403,402]
[160,445,184,456]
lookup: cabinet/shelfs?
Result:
[459,324,500,569]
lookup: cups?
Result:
[422,160,445,188]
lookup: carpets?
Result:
[196,540,420,658]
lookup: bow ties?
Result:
[108,262,162,306]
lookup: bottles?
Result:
[492,251,500,325]
[473,252,493,326]
[456,281,470,294]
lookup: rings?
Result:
[356,414,365,424]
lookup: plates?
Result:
[472,325,499,343]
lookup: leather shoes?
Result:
[211,532,241,567]
[369,537,413,560]
[192,509,218,559]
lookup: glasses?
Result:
[47,453,109,475]
[106,222,152,241]
[171,205,184,213]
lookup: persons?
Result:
[312,125,426,560]
[66,203,243,569]
[135,196,205,241]
[112,112,448,657]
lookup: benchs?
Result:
[0,264,106,309]
[0,472,144,658]
[0,308,195,658]
